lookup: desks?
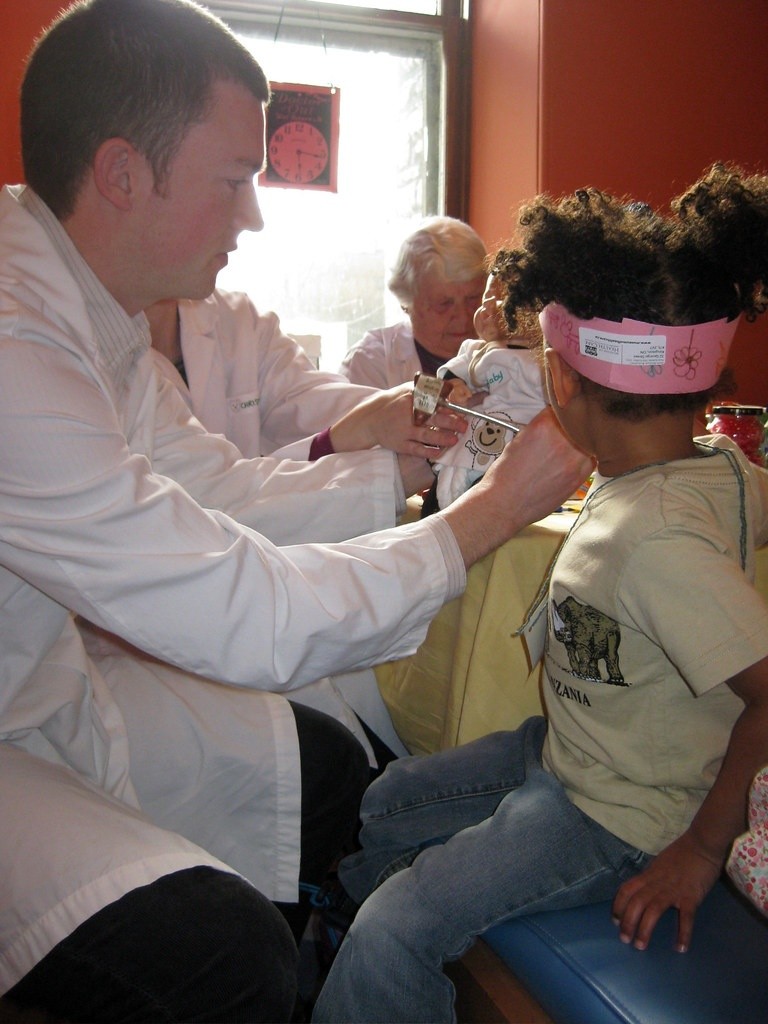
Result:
[375,485,582,757]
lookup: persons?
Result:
[313,172,768,1024]
[430,250,550,511]
[333,211,493,425]
[0,0,597,1024]
[82,288,466,904]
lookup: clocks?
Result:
[266,120,329,187]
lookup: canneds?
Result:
[712,406,766,465]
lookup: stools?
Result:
[412,832,768,1024]
